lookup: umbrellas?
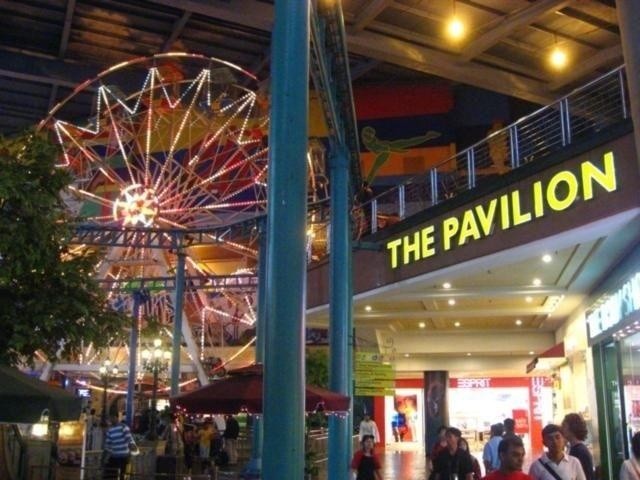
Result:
[0,359,82,425]
[177,362,350,420]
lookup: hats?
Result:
[359,434,375,444]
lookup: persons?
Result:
[358,413,381,449]
[422,412,640,479]
[86,403,240,480]
[351,435,384,480]
